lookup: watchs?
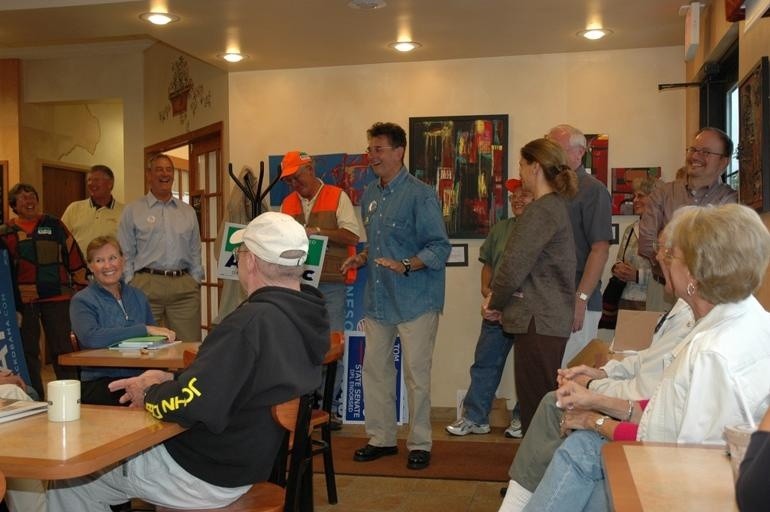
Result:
[576,291,590,301]
[401,258,411,276]
[595,416,610,439]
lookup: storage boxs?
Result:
[568,338,624,372]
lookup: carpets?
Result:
[282,432,521,482]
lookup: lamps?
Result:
[388,42,422,52]
[576,28,614,41]
[137,12,180,26]
[215,53,250,63]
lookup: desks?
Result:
[59,342,202,370]
[601,440,741,512]
[0,399,192,482]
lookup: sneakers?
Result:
[445,416,492,436]
[504,418,524,438]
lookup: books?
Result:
[109,336,182,351]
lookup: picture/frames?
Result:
[608,224,619,244]
[445,244,468,267]
[189,190,207,242]
[409,114,509,240]
[739,56,770,213]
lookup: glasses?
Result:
[685,146,724,158]
[232,247,249,261]
[652,239,662,253]
[662,248,682,265]
[364,146,390,153]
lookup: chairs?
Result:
[69,330,79,352]
[158,394,311,512]
[310,330,345,505]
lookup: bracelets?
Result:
[316,227,320,235]
[627,399,633,421]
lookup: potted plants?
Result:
[168,56,195,117]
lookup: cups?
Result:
[724,422,760,488]
[46,379,81,423]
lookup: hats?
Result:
[505,179,523,193]
[229,211,310,266]
[280,151,313,182]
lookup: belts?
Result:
[136,266,187,277]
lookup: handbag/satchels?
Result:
[602,276,626,304]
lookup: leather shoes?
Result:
[354,444,398,461]
[407,450,430,469]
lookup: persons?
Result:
[0,369,46,512]
[482,138,577,495]
[500,222,691,511]
[340,122,450,468]
[0,183,94,402]
[47,211,330,512]
[611,179,666,310]
[522,205,770,512]
[638,127,738,312]
[279,152,360,431]
[60,166,126,273]
[69,236,177,406]
[446,178,535,439]
[116,154,202,342]
[548,125,612,333]
